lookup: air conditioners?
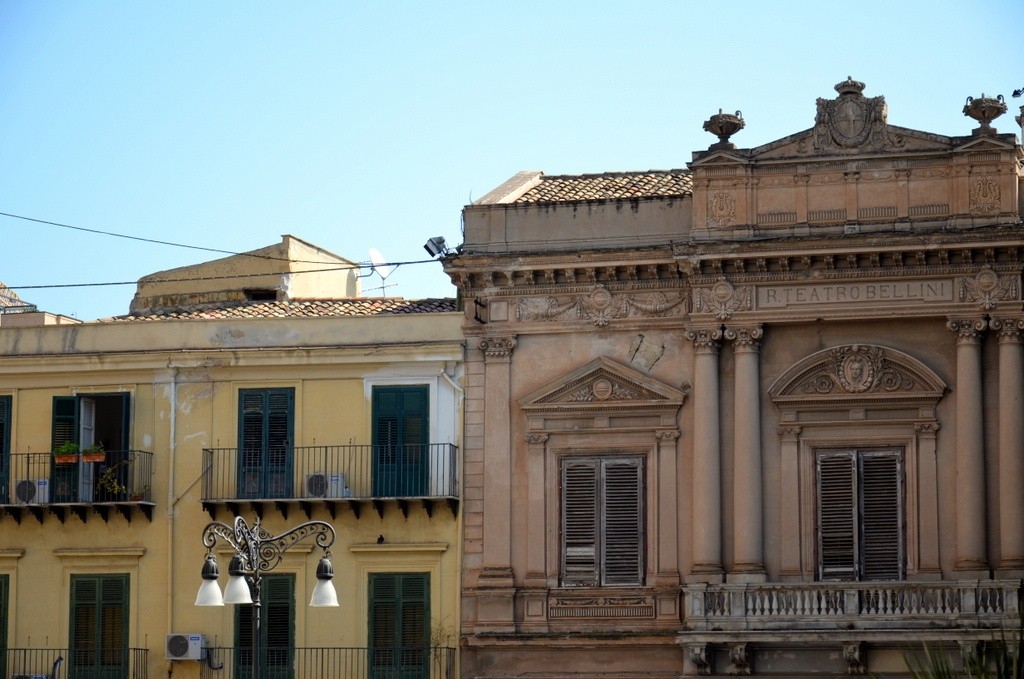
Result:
[14,479,49,505]
[164,633,208,660]
[305,472,346,498]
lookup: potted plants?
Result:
[52,441,79,464]
[82,443,106,462]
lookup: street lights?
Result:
[193,516,339,679]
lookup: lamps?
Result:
[424,237,448,257]
